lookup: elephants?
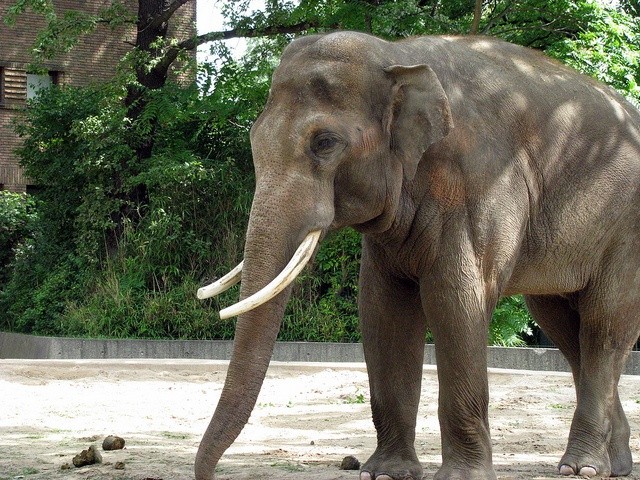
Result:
[194,29,640,480]
